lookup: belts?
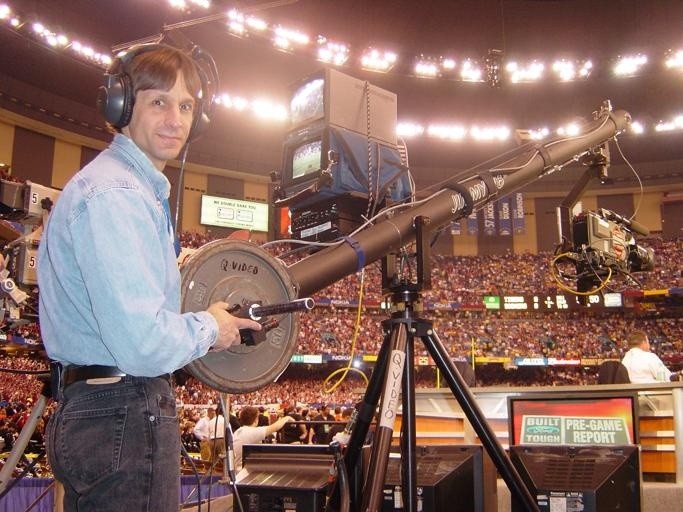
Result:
[58,364,173,386]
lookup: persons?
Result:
[0,162,59,481]
[34,43,263,511]
[173,227,683,492]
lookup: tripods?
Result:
[327,311,541,512]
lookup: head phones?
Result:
[102,46,216,143]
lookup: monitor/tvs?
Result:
[236,443,369,512]
[372,444,485,512]
[505,391,640,446]
[511,445,642,512]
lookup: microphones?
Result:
[191,41,228,115]
